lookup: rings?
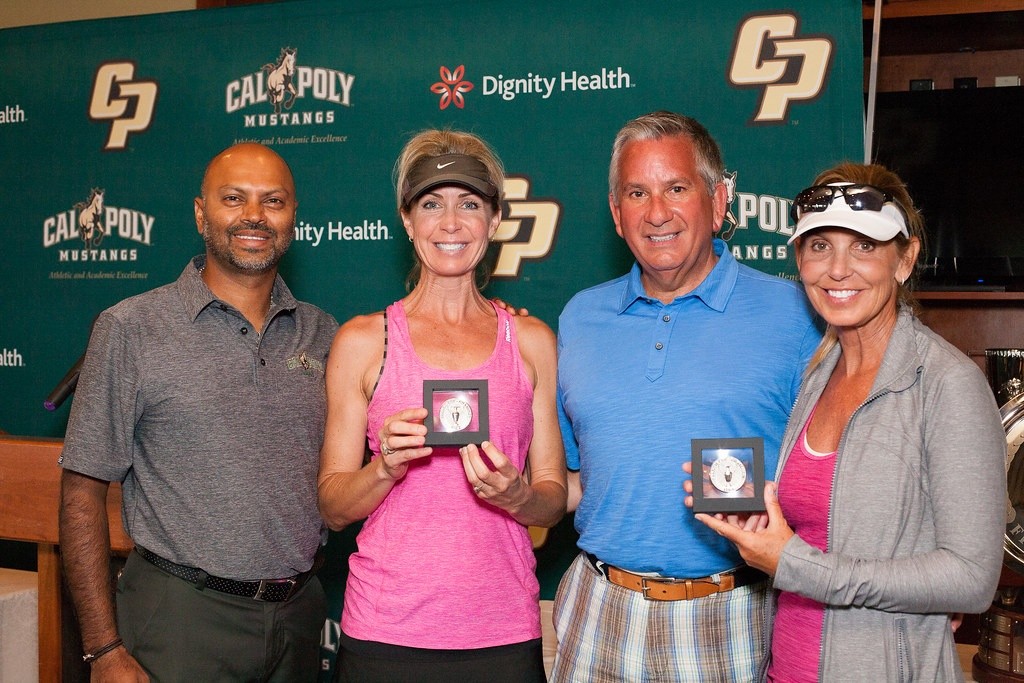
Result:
[379,439,395,455]
[472,484,484,493]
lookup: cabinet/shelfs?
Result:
[858,0,1024,385]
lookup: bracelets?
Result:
[82,640,124,663]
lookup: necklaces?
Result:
[196,262,274,340]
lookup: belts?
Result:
[136,543,323,602]
[587,552,769,602]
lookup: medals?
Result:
[710,457,746,493]
[440,398,472,432]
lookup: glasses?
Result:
[790,185,907,217]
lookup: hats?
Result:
[785,183,908,244]
[404,154,500,205]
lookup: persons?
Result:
[682,163,1008,683]
[56,142,532,683]
[319,127,568,683]
[557,106,964,682]
